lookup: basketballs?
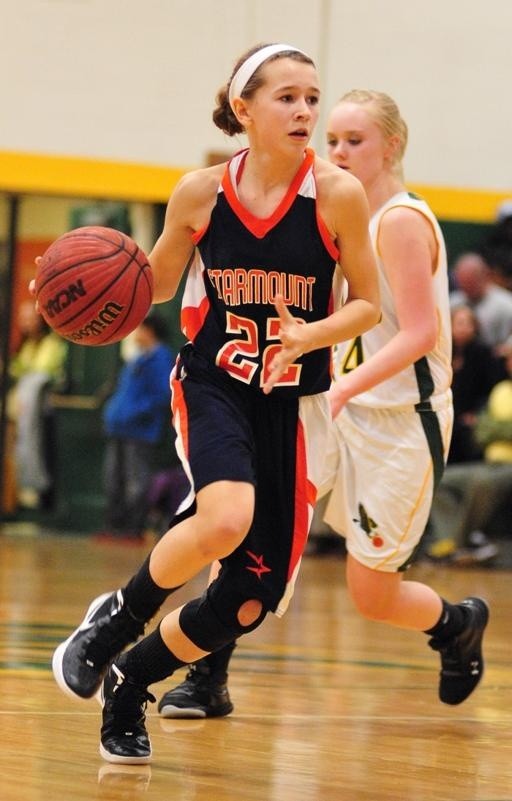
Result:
[36,226,154,345]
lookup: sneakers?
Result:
[428,595,490,705]
[157,665,233,719]
[51,588,156,766]
